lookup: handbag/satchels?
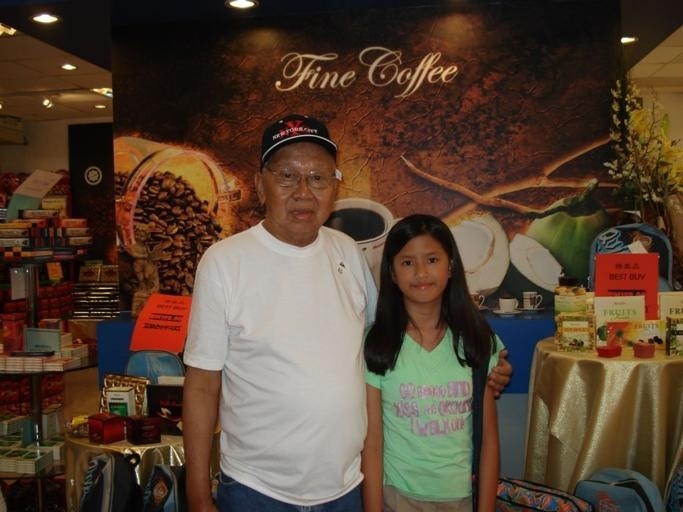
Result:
[473,466,683,512]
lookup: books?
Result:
[0,403,64,475]
[67,260,121,321]
[0,317,103,373]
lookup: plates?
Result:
[493,310,522,314]
[518,308,545,310]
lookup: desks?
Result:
[94,315,138,391]
[65,425,186,512]
[484,307,554,394]
[523,337,683,496]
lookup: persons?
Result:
[181,110,512,512]
[360,212,507,512]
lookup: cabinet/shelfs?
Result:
[0,255,99,512]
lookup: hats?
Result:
[260,115,337,168]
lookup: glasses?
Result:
[263,166,339,190]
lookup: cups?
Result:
[470,293,485,307]
[499,297,519,312]
[322,198,404,275]
[523,292,542,308]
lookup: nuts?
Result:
[113,170,224,295]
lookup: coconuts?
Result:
[509,232,562,306]
[525,177,615,284]
[440,209,509,299]
